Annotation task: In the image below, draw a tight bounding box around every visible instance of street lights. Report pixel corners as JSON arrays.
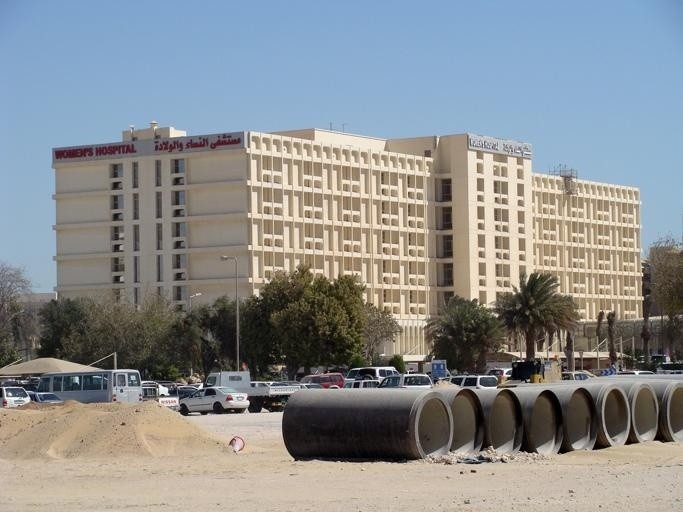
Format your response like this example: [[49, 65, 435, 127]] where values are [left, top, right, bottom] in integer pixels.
[[189, 291, 202, 311], [219, 254, 241, 372], [578, 350, 583, 371]]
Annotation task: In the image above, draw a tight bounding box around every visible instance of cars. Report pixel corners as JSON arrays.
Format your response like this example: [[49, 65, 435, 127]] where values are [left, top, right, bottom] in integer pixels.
[[178, 385, 251, 416]]
